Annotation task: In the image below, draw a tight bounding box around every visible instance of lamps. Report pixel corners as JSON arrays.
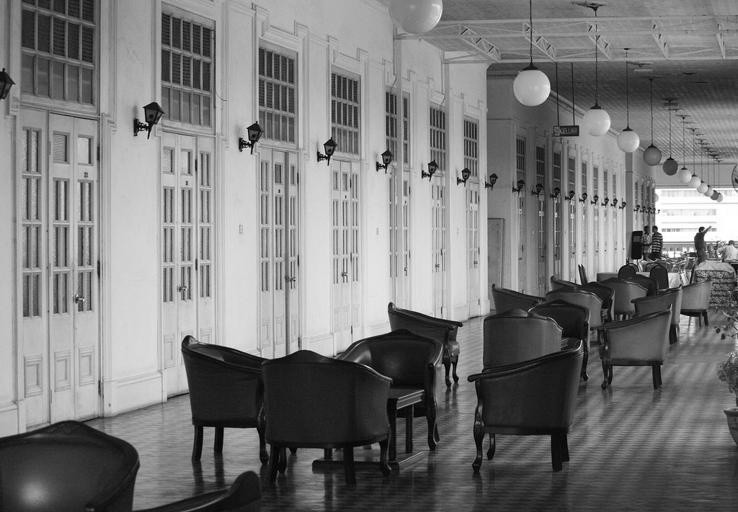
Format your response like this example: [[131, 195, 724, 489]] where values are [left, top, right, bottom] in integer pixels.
[[237, 121, 265, 157], [316, 137, 338, 167], [616, 51, 724, 203], [387, 1, 445, 35], [375, 150, 394, 175], [420, 159, 439, 183], [0, 68, 16, 101], [512, 0, 552, 109], [132, 101, 165, 141], [583, 9, 612, 139], [456, 167, 656, 216]]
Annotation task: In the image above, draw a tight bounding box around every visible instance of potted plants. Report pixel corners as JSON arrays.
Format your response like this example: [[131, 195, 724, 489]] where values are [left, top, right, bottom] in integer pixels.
[[713, 285, 737, 448]]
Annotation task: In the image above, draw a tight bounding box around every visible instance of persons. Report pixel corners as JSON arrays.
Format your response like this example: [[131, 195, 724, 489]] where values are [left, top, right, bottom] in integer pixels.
[[722, 240, 738, 262], [651, 226, 663, 260], [694, 226, 712, 263], [639, 226, 653, 260]]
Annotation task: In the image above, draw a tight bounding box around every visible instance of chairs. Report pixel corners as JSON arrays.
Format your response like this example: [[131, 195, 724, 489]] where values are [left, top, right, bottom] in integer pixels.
[[141, 469, 264, 512], [0, 416, 140, 512]]
